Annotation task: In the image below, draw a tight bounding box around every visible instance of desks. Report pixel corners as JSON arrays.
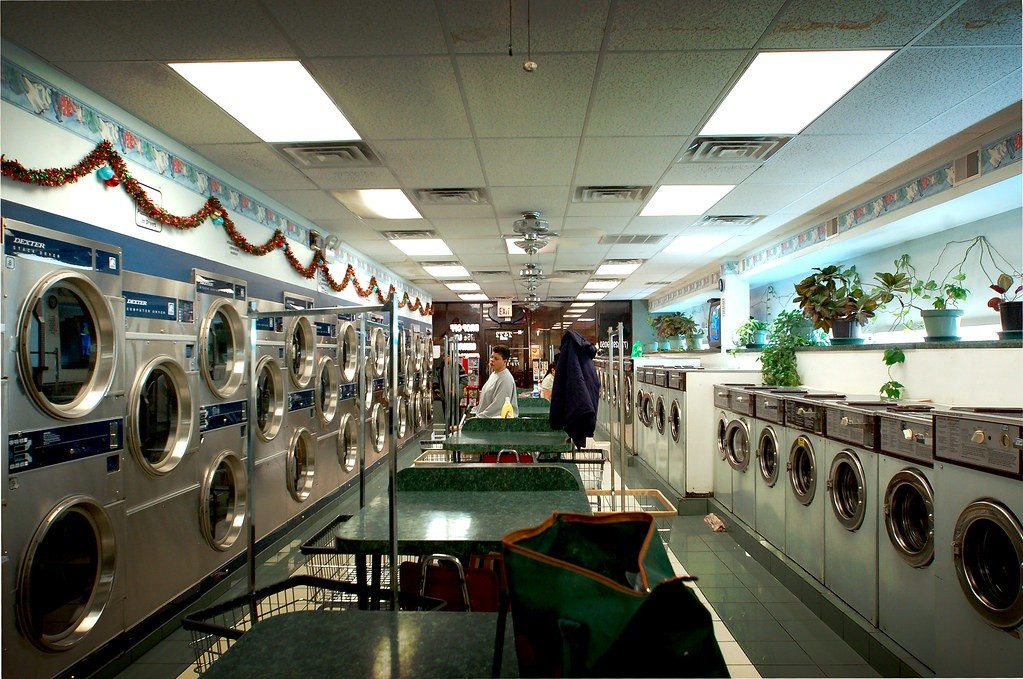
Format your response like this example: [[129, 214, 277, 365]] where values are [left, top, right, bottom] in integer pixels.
[[197, 610, 521, 679], [517, 398, 551, 419], [443, 418, 572, 463], [335, 463, 593, 611]]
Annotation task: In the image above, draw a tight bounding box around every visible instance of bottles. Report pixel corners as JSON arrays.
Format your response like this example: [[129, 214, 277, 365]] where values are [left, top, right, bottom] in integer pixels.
[[631, 341, 642, 357], [532, 387, 539, 398], [501, 397, 515, 418]]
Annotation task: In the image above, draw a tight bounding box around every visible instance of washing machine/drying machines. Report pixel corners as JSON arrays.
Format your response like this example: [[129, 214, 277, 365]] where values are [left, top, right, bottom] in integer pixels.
[[283, 291, 322, 528], [669, 370, 687, 497], [1, 216, 123, 679], [122, 271, 201, 637], [322, 307, 340, 504], [933, 405, 1023, 678], [713, 384, 743, 514], [247, 296, 288, 545], [823, 403, 915, 629], [644, 367, 655, 472], [755, 393, 805, 556], [592, 358, 632, 455], [340, 320, 361, 487], [656, 368, 668, 483], [783, 398, 834, 586], [729, 389, 774, 532], [876, 411, 932, 672], [360, 321, 432, 475], [636, 367, 644, 462], [192, 267, 248, 577]]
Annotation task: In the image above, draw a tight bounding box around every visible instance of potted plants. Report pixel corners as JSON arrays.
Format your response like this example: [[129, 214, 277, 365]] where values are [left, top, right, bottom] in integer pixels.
[[646, 312, 705, 352], [755, 309, 829, 389], [793, 265, 912, 338], [920, 284, 967, 337], [987, 274, 1023, 330], [728, 318, 773, 358]]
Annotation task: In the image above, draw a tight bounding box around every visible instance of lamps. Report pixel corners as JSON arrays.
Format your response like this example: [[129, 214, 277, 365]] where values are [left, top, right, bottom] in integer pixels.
[[523, 293, 540, 312], [518, 263, 543, 292], [512, 211, 559, 256], [321, 235, 344, 264]]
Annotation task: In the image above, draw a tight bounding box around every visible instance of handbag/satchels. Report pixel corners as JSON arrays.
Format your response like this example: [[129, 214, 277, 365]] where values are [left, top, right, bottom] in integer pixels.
[[501, 511, 729, 679]]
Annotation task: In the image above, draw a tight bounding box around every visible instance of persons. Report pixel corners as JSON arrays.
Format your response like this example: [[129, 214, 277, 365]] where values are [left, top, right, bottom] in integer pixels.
[[475, 345, 520, 419], [433, 350, 469, 435], [540, 361, 556, 403]]
